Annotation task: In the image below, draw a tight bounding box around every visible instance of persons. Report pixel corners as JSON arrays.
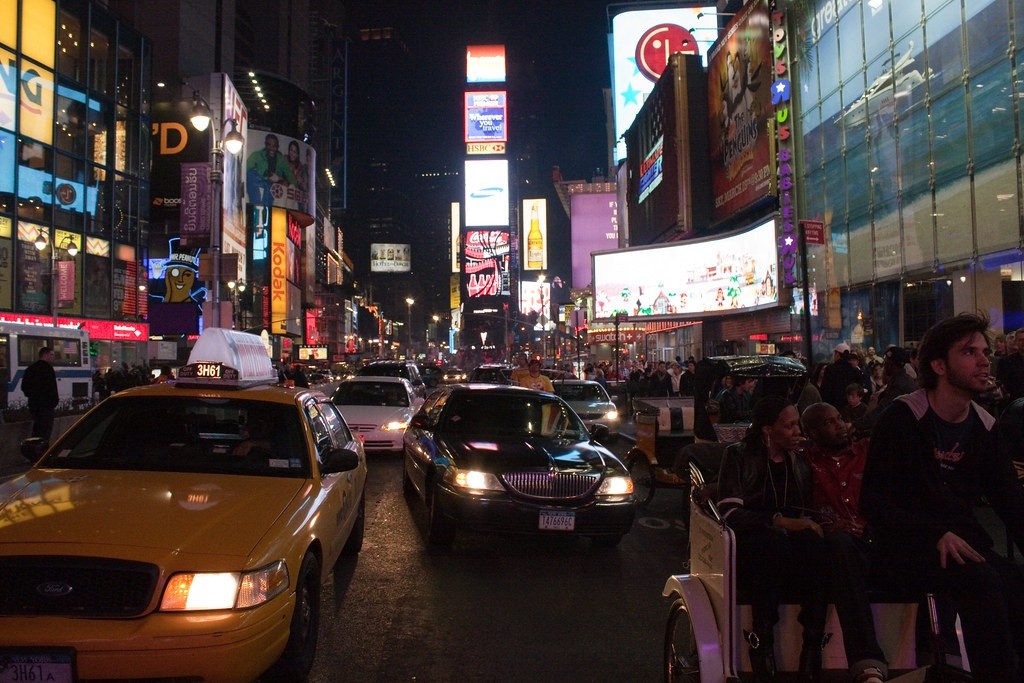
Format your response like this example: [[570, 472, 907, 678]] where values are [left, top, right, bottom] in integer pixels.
[[21, 347, 59, 441], [858, 313, 1024, 683], [512, 353, 555, 394], [716, 398, 890, 683], [585, 356, 705, 400], [716, 324, 1024, 427], [231, 405, 299, 457], [92, 362, 174, 392], [247, 134, 311, 192], [293, 365, 309, 388]]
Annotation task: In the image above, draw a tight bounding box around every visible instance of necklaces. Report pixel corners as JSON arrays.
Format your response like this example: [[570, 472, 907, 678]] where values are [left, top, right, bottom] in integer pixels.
[[768, 455, 788, 506], [530, 373, 540, 378]]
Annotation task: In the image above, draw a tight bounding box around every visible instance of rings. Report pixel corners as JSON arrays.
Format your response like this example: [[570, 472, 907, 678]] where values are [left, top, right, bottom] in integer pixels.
[[851, 528, 855, 532]]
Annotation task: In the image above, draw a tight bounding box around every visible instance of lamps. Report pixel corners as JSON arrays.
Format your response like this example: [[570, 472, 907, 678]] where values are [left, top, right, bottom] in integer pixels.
[[682, 39, 715, 46], [698, 12, 736, 20], [689, 28, 725, 34]]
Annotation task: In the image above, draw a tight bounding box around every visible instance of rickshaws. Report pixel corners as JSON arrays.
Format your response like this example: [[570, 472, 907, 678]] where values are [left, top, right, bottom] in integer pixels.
[[623, 394, 695, 506], [661, 443, 974, 683]]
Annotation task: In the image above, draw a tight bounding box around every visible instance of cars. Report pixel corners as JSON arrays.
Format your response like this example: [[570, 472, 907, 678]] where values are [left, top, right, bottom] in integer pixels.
[[328, 375, 426, 453], [284, 359, 370, 399], [548, 380, 621, 444], [415, 359, 470, 389], [402, 381, 636, 555], [0, 384, 368, 683]]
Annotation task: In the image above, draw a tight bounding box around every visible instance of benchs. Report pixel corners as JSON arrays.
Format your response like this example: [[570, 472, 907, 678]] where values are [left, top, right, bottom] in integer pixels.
[[631, 396, 695, 445], [681, 442, 881, 607]]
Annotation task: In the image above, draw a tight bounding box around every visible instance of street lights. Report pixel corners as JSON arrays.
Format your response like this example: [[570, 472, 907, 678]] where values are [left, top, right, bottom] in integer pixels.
[[35, 227, 78, 326], [188, 89, 246, 328], [405, 298, 415, 359]]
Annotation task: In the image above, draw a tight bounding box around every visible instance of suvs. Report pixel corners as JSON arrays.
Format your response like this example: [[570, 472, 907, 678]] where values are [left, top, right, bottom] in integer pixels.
[[470, 362, 515, 384], [356, 358, 426, 398]]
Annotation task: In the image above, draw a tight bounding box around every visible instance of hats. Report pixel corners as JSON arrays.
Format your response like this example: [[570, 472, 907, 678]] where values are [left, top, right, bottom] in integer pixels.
[[830, 343, 851, 354]]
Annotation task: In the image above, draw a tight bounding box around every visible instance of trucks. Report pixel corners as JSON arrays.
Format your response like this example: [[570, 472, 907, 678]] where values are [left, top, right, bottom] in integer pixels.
[[0, 322, 91, 415]]
